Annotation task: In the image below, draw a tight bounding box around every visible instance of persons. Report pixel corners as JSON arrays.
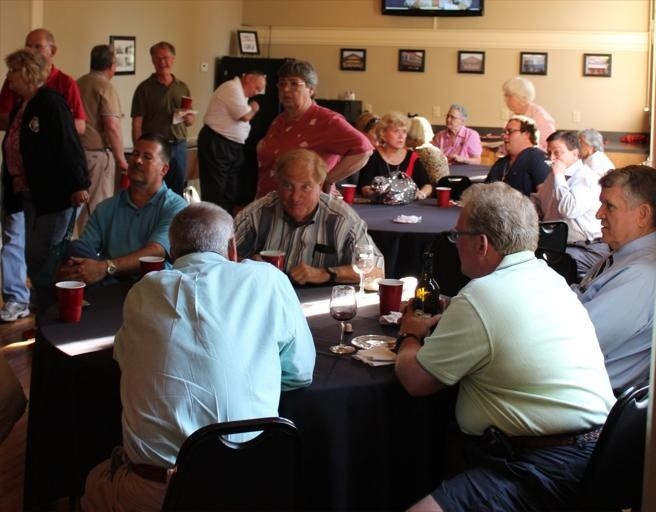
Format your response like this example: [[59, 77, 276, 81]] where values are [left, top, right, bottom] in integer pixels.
[[345, 76, 617, 282], [255, 56, 376, 204], [59, 131, 189, 319], [229, 150, 385, 285], [0, 26, 136, 323], [388, 181, 620, 509], [577, 166, 656, 398], [402, 1, 474, 13], [130, 42, 192, 198], [81, 202, 314, 511], [196, 71, 269, 213]]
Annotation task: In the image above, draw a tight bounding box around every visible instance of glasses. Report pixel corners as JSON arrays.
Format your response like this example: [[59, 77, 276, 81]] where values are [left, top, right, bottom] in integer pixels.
[[506, 128, 520, 135], [275, 80, 305, 89], [8, 67, 20, 73], [447, 229, 471, 243]]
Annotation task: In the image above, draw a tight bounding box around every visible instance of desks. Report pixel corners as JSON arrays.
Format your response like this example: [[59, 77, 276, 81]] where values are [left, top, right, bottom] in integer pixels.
[[31, 281, 133, 512], [340, 192, 466, 283], [448, 161, 493, 180], [280, 285, 453, 512]]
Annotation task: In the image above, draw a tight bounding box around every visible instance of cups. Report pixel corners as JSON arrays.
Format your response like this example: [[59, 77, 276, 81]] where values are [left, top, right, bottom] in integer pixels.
[[378, 277, 404, 319], [120, 171, 129, 189], [180, 96, 192, 111], [435, 186, 452, 207], [260, 249, 286, 273], [54, 280, 86, 323], [139, 256, 165, 277], [341, 183, 357, 206]]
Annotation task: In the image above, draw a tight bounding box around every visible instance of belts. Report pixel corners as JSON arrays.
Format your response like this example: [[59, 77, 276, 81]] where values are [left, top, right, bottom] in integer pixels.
[[512, 429, 599, 447], [130, 465, 166, 483]]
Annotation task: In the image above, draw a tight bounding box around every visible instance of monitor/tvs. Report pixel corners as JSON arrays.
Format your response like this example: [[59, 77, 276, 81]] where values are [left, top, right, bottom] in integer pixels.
[[380, 0, 485, 18]]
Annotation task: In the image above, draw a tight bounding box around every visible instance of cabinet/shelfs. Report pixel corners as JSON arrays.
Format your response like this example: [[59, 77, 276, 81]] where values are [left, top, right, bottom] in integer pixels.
[[214, 56, 365, 157]]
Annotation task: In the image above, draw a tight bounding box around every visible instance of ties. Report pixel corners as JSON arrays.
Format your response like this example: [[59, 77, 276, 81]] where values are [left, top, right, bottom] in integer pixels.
[[592, 256, 613, 278]]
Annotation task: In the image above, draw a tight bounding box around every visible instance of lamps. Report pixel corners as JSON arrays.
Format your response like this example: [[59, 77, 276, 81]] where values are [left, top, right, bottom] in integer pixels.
[[397, 48, 426, 72]]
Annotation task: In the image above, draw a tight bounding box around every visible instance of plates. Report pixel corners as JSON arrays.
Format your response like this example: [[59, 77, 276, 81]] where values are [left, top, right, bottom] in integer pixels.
[[352, 333, 398, 350]]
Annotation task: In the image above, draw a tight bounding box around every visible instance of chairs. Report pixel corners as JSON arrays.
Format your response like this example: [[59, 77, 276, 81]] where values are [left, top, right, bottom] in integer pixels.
[[433, 173, 474, 202], [535, 219, 581, 284], [566, 374, 650, 510], [161, 416, 316, 512]]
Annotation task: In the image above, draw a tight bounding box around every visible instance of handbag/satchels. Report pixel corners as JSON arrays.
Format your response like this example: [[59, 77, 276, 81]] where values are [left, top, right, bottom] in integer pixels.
[[46, 202, 90, 289], [369, 171, 418, 206]]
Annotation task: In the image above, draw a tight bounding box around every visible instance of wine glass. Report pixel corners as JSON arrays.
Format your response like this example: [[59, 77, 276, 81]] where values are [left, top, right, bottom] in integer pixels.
[[328, 284, 359, 356], [353, 242, 377, 301]]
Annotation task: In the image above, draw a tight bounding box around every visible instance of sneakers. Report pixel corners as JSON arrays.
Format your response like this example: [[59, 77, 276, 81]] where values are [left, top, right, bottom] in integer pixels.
[[0, 299, 30, 322]]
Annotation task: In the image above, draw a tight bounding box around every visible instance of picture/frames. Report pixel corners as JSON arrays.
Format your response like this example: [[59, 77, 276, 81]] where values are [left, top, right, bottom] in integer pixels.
[[109, 34, 137, 76], [457, 50, 486, 74], [582, 52, 612, 78], [235, 29, 259, 56], [519, 50, 548, 76], [339, 47, 367, 71]]
